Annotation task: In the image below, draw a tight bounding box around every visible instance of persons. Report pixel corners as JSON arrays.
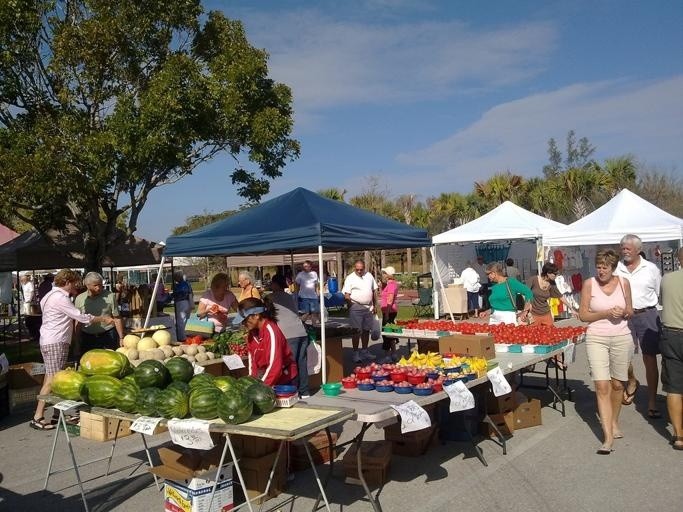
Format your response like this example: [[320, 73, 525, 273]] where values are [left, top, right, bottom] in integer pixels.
[[342, 260, 379, 363], [660, 247, 683, 450], [378, 266, 399, 364], [612, 234, 662, 418], [473, 256, 489, 311], [459, 261, 482, 318], [479, 261, 534, 325], [502, 258, 522, 281], [526, 262, 579, 360], [579, 249, 635, 454]]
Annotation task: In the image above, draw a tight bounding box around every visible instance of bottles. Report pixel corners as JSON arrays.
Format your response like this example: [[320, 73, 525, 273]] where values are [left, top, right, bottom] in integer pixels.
[[328, 277, 338, 294]]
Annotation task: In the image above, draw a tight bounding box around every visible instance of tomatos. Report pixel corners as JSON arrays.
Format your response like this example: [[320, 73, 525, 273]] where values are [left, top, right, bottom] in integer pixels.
[[397, 318, 586, 346]]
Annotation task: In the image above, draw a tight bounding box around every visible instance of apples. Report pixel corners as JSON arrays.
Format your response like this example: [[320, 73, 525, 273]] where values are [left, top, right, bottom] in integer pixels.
[[342, 362, 448, 390]]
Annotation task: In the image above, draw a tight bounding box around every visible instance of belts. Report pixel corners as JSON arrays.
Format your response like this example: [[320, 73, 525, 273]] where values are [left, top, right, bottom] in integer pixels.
[[633, 306, 656, 314]]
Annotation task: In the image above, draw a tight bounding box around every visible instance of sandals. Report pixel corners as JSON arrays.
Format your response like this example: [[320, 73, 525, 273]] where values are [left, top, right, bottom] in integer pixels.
[[669, 435, 683, 450], [29, 415, 60, 431]]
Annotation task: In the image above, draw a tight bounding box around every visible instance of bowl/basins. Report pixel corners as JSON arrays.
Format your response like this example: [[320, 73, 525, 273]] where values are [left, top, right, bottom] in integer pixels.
[[272, 385, 299, 393], [380, 318, 419, 334], [341, 352, 478, 396], [319, 383, 343, 396]]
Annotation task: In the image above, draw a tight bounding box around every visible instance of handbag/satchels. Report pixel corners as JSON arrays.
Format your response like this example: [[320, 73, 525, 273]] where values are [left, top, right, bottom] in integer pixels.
[[184, 312, 215, 338], [515, 309, 535, 326]]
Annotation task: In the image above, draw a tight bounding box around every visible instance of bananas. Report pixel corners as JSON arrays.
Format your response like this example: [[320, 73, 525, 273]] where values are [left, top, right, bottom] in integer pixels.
[[399, 351, 487, 372]]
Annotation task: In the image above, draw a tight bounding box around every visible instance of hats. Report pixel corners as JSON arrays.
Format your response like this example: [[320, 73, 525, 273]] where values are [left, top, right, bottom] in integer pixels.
[[232, 306, 265, 325], [381, 266, 396, 275]]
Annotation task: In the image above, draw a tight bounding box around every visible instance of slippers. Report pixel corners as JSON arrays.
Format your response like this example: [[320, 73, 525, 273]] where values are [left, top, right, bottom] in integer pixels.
[[613, 431, 625, 439], [648, 408, 663, 418], [622, 378, 640, 405], [596, 444, 612, 455]]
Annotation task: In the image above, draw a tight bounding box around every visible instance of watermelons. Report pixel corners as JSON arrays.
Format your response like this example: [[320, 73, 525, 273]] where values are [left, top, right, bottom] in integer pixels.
[[50, 349, 276, 425]]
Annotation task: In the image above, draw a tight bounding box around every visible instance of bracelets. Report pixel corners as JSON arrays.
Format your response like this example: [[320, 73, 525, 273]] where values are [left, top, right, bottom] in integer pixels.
[[570, 306, 574, 311], [523, 309, 529, 313]]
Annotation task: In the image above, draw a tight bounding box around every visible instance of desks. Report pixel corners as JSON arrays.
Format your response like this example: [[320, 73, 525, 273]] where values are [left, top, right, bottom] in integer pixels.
[[31, 304, 589, 511], [323, 291, 346, 312], [440, 287, 469, 321]]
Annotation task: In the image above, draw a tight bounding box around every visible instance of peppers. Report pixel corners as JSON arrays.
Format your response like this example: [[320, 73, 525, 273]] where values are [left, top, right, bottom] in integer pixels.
[[202, 331, 232, 359]]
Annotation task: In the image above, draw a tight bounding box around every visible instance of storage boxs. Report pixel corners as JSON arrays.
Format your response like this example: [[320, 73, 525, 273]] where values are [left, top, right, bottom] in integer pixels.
[[6, 361, 45, 407]]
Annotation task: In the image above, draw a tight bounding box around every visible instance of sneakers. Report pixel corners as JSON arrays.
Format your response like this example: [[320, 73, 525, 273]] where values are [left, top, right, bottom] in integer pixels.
[[352, 351, 362, 363], [359, 348, 377, 360], [377, 354, 400, 364]]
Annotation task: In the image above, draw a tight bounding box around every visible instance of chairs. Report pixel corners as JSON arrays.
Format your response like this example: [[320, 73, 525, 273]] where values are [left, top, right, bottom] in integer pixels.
[[412, 288, 433, 317]]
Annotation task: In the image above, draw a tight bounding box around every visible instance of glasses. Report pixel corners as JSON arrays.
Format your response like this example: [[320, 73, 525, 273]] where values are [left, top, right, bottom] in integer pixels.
[[355, 268, 364, 272]]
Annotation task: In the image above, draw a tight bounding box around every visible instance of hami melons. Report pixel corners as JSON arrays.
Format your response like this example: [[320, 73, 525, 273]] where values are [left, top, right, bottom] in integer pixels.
[[115, 330, 213, 367]]
